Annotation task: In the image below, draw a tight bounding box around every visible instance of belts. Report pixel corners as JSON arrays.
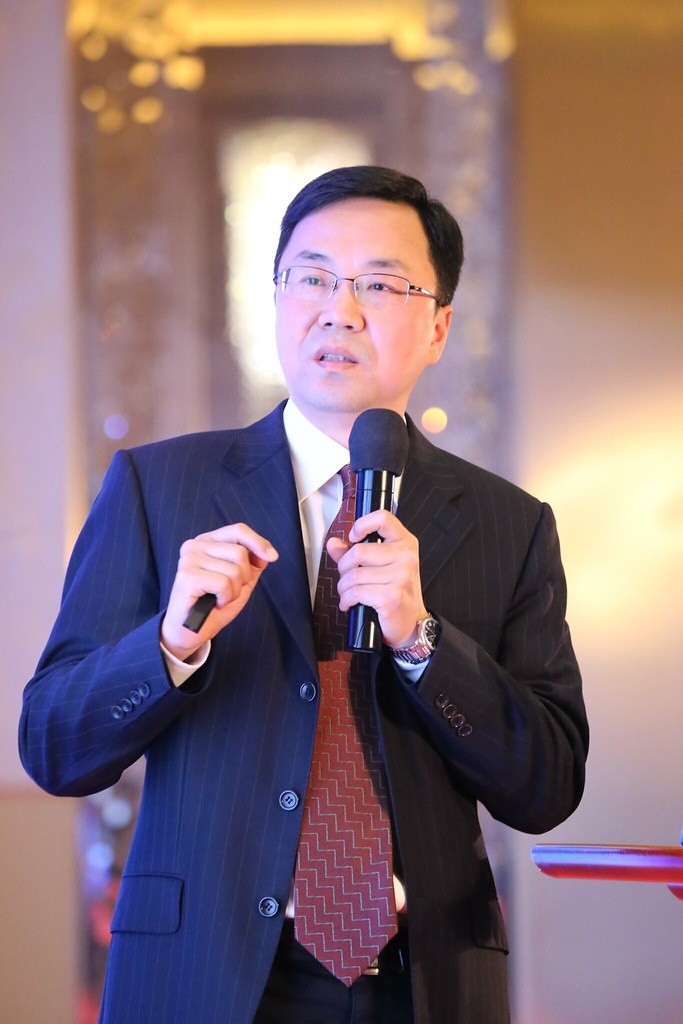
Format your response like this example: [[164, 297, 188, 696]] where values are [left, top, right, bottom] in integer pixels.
[[273, 934, 406, 977]]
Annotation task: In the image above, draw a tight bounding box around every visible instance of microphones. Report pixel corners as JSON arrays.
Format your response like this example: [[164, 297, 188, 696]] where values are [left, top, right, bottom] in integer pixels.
[[342, 408, 410, 652]]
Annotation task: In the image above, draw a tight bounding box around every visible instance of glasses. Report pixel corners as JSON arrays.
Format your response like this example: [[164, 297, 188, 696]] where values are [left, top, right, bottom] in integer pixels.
[[273, 265, 441, 310]]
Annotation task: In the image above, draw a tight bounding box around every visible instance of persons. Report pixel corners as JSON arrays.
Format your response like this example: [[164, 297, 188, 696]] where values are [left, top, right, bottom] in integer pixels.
[[17, 164, 590, 1024]]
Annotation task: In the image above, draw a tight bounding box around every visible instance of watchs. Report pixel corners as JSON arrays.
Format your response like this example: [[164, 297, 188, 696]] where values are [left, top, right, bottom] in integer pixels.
[[388, 613, 441, 665]]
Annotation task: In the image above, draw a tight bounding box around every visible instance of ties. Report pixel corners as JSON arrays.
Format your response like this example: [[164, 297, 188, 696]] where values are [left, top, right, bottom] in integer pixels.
[[293, 464, 398, 987]]
